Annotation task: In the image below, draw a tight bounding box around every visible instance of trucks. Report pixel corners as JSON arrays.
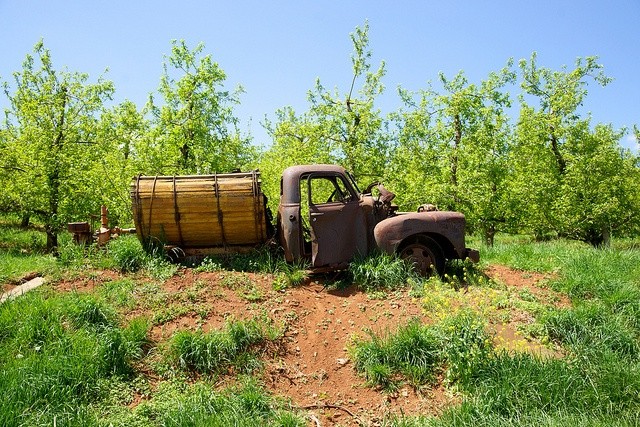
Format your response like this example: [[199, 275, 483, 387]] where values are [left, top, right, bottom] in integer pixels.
[[131, 163, 479, 282]]
